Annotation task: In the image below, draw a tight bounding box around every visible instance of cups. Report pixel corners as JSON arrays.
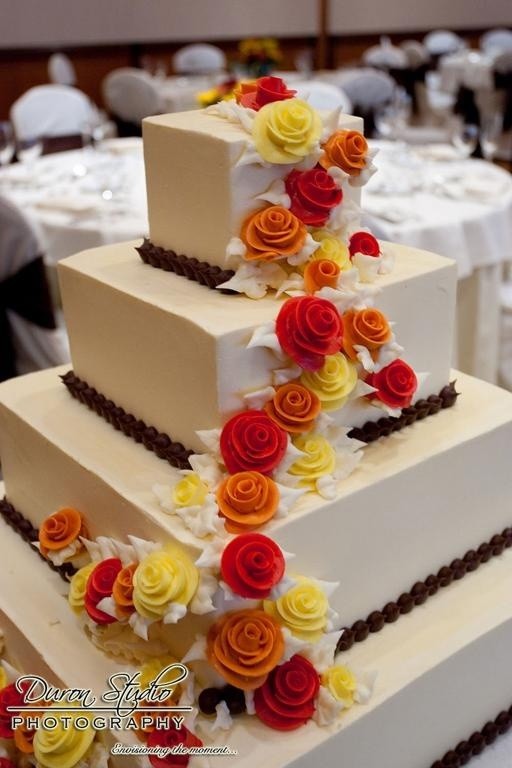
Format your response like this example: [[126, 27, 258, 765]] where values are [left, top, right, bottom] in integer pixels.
[[449, 124, 479, 160]]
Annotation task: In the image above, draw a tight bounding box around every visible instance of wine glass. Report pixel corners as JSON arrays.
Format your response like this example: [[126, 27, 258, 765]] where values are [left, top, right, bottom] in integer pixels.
[[0, 121, 46, 188]]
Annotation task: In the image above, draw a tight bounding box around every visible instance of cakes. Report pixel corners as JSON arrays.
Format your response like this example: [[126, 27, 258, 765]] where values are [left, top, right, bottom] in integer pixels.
[[0, 74, 512, 767]]
